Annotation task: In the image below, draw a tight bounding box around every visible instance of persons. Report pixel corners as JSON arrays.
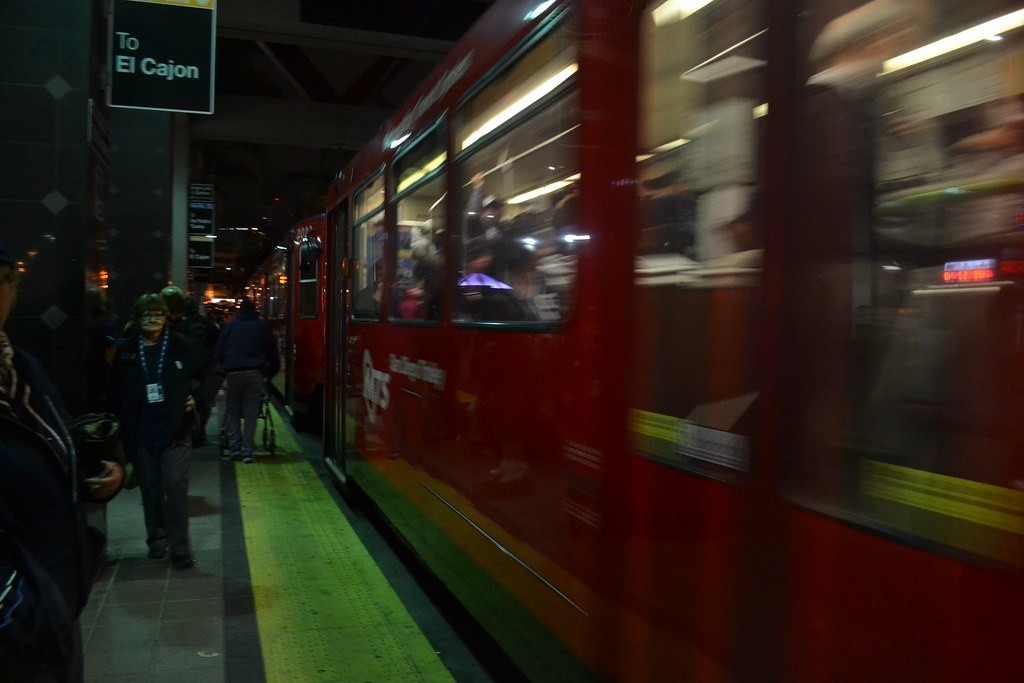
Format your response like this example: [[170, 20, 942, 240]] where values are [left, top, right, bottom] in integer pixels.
[[355, 170, 581, 319], [0, 246, 126, 683], [111, 285, 280, 567]]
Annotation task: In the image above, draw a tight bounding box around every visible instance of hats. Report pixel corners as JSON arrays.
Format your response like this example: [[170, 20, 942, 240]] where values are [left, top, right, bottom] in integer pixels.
[[240, 301, 255, 311], [0, 243, 14, 270]]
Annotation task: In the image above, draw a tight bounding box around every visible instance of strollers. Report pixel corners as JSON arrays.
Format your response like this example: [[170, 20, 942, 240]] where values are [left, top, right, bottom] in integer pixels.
[[220, 377, 276, 457]]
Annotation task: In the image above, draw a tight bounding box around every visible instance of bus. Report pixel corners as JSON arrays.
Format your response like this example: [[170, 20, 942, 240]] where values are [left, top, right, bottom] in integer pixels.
[[245, 1, 1024, 683]]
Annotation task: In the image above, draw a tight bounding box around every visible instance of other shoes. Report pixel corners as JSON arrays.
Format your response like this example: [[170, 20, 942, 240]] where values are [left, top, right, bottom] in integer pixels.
[[231, 446, 241, 458], [242, 451, 253, 462], [145, 542, 170, 563], [169, 549, 196, 566]]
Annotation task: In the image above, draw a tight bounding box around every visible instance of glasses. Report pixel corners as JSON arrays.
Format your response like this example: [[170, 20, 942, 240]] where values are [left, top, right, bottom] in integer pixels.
[[140, 313, 166, 320], [0, 270, 16, 284]]
